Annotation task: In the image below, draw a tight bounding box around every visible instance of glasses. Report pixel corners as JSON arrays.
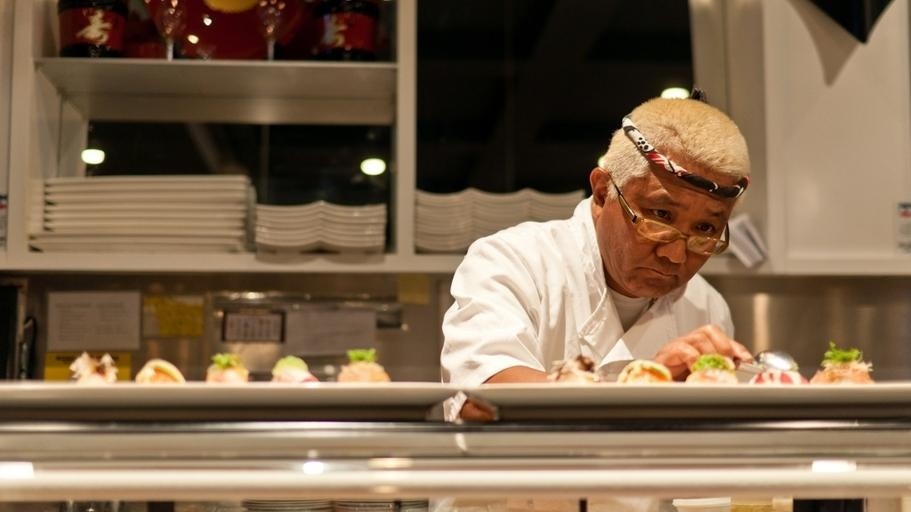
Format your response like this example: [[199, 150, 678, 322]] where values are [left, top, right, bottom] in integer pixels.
[[606, 172, 730, 256]]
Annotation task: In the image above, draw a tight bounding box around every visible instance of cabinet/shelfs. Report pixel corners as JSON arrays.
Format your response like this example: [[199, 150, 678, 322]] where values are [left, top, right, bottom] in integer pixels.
[[0, 1, 911, 278]]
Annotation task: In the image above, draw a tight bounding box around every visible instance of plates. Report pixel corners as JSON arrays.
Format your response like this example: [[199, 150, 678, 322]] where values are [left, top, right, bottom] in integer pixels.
[[454, 382, 911, 425], [413, 185, 585, 255], [26, 174, 254, 254], [0, 382, 461, 423], [251, 200, 388, 257]]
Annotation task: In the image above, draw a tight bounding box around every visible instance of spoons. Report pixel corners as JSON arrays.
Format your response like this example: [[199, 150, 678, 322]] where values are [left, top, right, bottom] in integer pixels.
[[735, 349, 794, 371]]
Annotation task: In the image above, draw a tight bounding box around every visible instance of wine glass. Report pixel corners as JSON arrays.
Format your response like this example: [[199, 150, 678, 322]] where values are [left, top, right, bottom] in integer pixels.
[[257, 0, 287, 61], [153, 0, 188, 60]]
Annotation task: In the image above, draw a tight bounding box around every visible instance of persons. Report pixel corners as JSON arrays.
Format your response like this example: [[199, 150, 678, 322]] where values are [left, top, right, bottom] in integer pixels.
[[438, 96, 753, 420]]
